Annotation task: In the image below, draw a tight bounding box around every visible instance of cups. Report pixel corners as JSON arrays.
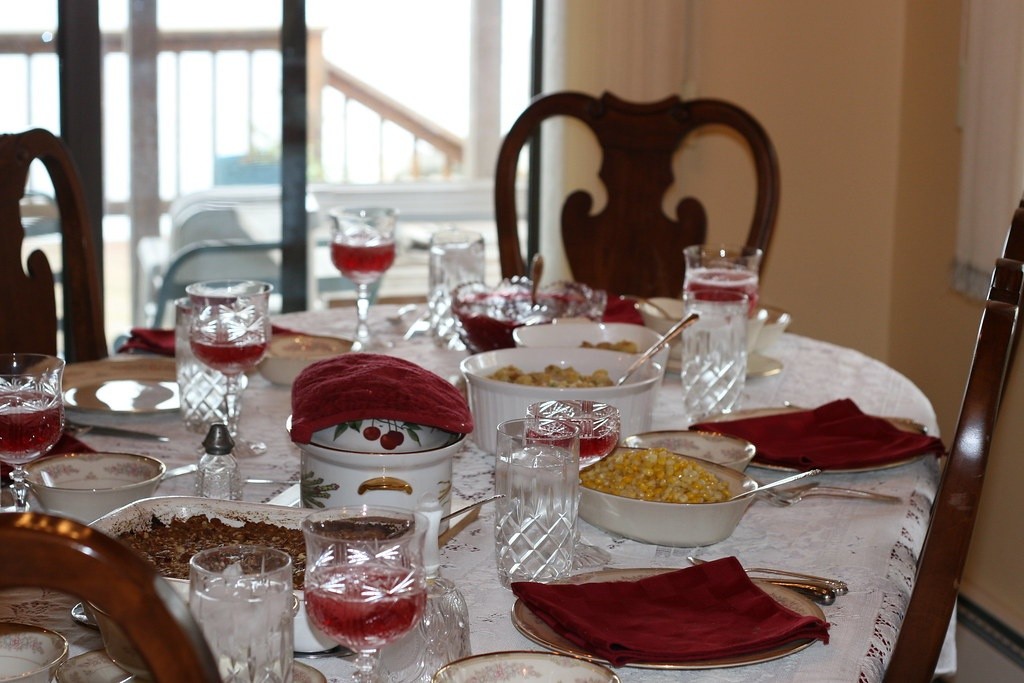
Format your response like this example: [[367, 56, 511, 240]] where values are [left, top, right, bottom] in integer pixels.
[[494, 415, 580, 590], [682, 244, 763, 325], [175, 301, 246, 437], [187, 544, 296, 683], [680, 287, 749, 426], [426, 230, 485, 355]]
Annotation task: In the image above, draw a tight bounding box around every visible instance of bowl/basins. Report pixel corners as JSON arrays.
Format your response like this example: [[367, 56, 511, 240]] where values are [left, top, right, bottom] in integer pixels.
[[84, 577, 190, 683], [619, 429, 757, 474], [0, 621, 70, 683], [512, 320, 670, 372], [449, 280, 608, 354], [284, 412, 470, 537], [578, 445, 758, 548], [21, 452, 167, 523], [640, 298, 791, 379], [459, 346, 664, 456], [257, 333, 354, 386]]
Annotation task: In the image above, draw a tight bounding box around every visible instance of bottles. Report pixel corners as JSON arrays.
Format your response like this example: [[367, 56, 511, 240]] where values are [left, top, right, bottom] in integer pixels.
[[193, 422, 244, 502], [367, 489, 472, 683]]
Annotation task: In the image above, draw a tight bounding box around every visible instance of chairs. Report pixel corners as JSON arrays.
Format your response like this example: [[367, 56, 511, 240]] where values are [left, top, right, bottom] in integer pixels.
[[493, 87, 789, 307], [0, 127, 109, 363], [131, 184, 283, 329], [880, 195, 1024, 683], [0, 513, 212, 683]]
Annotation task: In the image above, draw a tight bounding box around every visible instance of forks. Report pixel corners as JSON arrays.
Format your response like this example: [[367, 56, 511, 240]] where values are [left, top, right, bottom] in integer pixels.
[[748, 475, 902, 505]]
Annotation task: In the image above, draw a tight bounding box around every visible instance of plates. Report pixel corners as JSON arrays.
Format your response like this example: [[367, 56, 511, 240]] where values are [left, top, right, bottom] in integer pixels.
[[699, 406, 929, 475], [63, 379, 186, 438], [85, 494, 313, 592], [54, 648, 150, 683], [511, 566, 827, 670], [432, 649, 620, 683]]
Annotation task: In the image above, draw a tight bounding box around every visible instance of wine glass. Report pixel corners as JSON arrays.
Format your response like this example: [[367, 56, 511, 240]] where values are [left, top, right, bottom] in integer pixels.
[[185, 280, 275, 456], [331, 221, 398, 355], [522, 400, 621, 568], [0, 349, 66, 513], [301, 505, 428, 683]]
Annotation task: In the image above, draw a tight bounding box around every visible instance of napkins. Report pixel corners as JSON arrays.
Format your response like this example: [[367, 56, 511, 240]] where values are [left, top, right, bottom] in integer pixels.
[[512, 554, 835, 668], [293, 350, 474, 441], [117, 326, 283, 357], [692, 396, 945, 469]]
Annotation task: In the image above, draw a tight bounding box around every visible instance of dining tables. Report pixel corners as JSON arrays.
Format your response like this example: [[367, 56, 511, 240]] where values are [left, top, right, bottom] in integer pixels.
[[0, 303, 960, 683]]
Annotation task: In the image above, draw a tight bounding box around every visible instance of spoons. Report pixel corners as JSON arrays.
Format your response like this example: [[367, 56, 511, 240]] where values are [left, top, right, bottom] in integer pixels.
[[687, 555, 849, 597]]
